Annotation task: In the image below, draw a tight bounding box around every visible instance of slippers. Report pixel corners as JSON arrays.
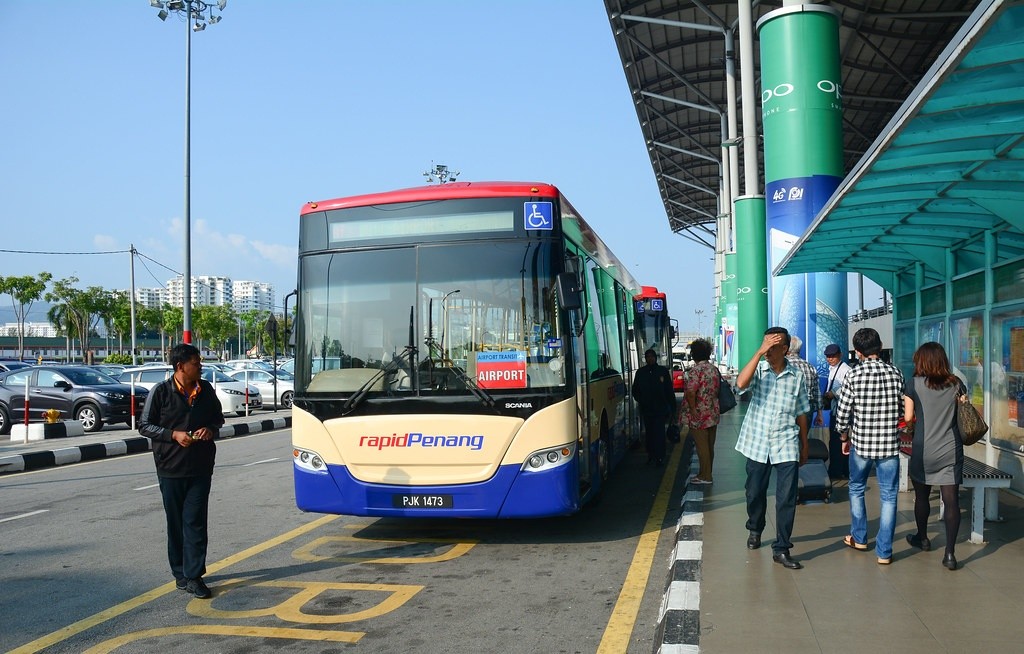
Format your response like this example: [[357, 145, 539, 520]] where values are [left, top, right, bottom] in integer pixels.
[[691, 477, 713, 484]]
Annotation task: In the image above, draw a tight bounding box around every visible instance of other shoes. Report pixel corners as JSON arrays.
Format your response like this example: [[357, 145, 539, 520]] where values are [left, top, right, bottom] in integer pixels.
[[878, 556, 892, 564], [844, 535, 867, 550], [657, 456, 664, 465]]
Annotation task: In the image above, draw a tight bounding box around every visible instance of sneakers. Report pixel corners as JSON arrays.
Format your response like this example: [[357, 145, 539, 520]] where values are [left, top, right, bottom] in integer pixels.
[[176, 577, 188, 590], [187, 577, 211, 598]]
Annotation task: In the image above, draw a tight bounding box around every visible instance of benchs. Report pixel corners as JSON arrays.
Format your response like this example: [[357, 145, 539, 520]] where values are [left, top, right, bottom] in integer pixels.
[[898, 447, 1013, 545]]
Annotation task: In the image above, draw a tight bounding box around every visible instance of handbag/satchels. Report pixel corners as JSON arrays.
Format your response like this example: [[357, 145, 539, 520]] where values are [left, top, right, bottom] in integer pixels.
[[955, 380, 988, 446], [822, 395, 832, 410], [716, 367, 737, 414], [667, 416, 681, 443]]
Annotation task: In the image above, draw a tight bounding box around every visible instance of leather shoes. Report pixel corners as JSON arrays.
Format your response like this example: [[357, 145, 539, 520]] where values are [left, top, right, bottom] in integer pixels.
[[773, 552, 801, 569], [906, 533, 931, 550], [747, 531, 761, 549], [943, 553, 957, 570]]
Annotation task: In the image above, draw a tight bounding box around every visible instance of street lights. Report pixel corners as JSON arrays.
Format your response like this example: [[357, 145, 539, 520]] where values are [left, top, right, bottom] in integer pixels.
[[147, 0, 226, 349]]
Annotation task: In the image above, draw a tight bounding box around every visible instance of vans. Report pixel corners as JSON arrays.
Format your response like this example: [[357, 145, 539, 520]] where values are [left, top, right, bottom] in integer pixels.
[[275, 356, 342, 386]]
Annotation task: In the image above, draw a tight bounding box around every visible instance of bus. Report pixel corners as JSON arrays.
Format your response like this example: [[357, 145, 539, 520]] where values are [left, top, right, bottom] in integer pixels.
[[263, 179, 646, 522], [633, 286, 680, 424]]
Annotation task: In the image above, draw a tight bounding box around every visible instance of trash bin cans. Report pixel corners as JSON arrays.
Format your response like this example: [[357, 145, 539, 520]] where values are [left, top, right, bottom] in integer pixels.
[[87, 351, 95, 365], [1016, 393, 1024, 428], [34, 352, 40, 359], [730, 374, 747, 402]]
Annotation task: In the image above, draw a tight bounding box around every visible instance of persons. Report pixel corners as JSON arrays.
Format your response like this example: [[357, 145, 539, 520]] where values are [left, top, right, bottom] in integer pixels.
[[139, 344, 225, 597], [991, 352, 1006, 385], [734, 327, 810, 569], [824, 344, 853, 479], [972, 351, 983, 386], [786, 336, 823, 434], [904, 342, 968, 570], [632, 349, 677, 466], [835, 328, 906, 563], [678, 338, 720, 484]]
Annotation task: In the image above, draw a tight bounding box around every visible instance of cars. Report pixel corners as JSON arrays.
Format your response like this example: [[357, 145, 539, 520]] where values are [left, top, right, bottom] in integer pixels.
[[0, 364, 149, 436], [670, 339, 694, 392], [0, 356, 296, 425]]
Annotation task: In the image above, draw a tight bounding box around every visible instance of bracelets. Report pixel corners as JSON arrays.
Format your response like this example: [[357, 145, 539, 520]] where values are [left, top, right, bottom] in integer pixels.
[[841, 436, 849, 442]]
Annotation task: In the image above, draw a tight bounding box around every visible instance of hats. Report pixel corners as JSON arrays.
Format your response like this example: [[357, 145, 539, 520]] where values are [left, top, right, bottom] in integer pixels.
[[824, 344, 841, 356]]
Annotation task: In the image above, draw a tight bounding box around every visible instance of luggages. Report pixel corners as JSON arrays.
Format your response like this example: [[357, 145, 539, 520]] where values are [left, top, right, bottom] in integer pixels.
[[796, 458, 832, 503]]
